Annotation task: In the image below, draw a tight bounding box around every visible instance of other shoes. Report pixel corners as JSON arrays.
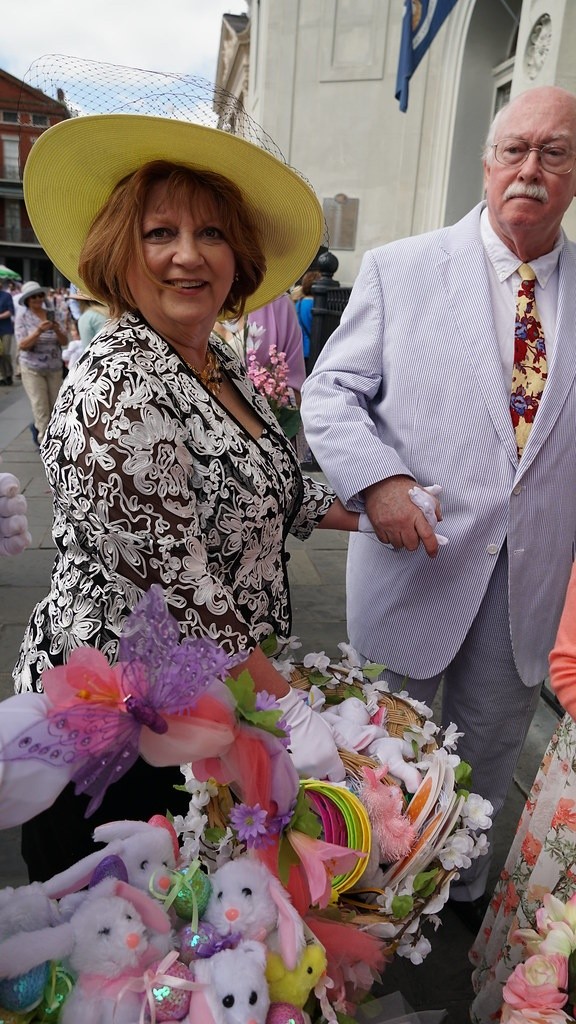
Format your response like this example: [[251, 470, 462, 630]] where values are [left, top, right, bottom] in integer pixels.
[[0, 380, 13, 386], [30, 423, 40, 446], [15, 373, 21, 377], [444, 890, 492, 939]]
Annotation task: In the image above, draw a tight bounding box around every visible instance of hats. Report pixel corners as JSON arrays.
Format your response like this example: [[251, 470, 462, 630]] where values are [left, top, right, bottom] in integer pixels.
[[65, 289, 96, 300], [18, 281, 48, 305], [23, 115, 323, 321]]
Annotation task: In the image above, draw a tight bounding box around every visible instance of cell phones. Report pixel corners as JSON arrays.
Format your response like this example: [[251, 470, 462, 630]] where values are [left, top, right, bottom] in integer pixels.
[[46, 310, 55, 323]]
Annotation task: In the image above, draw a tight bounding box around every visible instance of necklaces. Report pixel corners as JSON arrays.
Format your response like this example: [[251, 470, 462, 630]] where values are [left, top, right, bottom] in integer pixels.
[[182, 344, 224, 397]]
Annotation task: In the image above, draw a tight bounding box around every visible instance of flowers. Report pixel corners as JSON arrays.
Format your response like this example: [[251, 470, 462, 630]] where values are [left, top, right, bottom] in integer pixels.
[[214, 315, 300, 418], [173, 633, 494, 967]]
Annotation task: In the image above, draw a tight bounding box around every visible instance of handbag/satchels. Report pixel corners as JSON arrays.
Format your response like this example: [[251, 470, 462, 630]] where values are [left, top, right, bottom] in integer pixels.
[[63, 361, 69, 379]]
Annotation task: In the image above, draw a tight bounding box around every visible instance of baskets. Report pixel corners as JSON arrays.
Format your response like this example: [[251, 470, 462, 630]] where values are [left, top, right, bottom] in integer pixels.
[[202, 672, 468, 956]]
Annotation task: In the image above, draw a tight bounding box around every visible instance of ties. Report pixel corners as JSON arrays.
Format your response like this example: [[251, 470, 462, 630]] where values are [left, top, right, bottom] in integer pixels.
[[510, 263, 548, 462]]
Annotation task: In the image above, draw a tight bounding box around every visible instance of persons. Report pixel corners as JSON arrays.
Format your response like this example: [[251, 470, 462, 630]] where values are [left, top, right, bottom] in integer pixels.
[[0, 277, 110, 449], [230, 271, 320, 458], [298, 85, 576, 932], [24, 114, 450, 889]]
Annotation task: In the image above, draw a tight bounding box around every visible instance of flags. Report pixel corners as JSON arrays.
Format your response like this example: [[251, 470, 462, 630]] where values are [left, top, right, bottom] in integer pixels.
[[395, 0, 458, 113]]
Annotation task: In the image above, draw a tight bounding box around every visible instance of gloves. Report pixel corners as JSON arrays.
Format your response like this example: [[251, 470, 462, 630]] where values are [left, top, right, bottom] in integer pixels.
[[276, 684, 346, 782], [0, 473, 31, 557], [358, 484, 448, 545]]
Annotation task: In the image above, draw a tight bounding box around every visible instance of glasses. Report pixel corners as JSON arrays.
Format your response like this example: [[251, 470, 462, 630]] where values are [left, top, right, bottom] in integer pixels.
[[30, 293, 44, 299], [490, 138, 576, 175]]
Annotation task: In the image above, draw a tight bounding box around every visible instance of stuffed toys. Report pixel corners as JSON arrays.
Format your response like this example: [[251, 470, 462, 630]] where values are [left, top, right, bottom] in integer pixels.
[[0, 820, 327, 1024], [322, 698, 422, 794]]
